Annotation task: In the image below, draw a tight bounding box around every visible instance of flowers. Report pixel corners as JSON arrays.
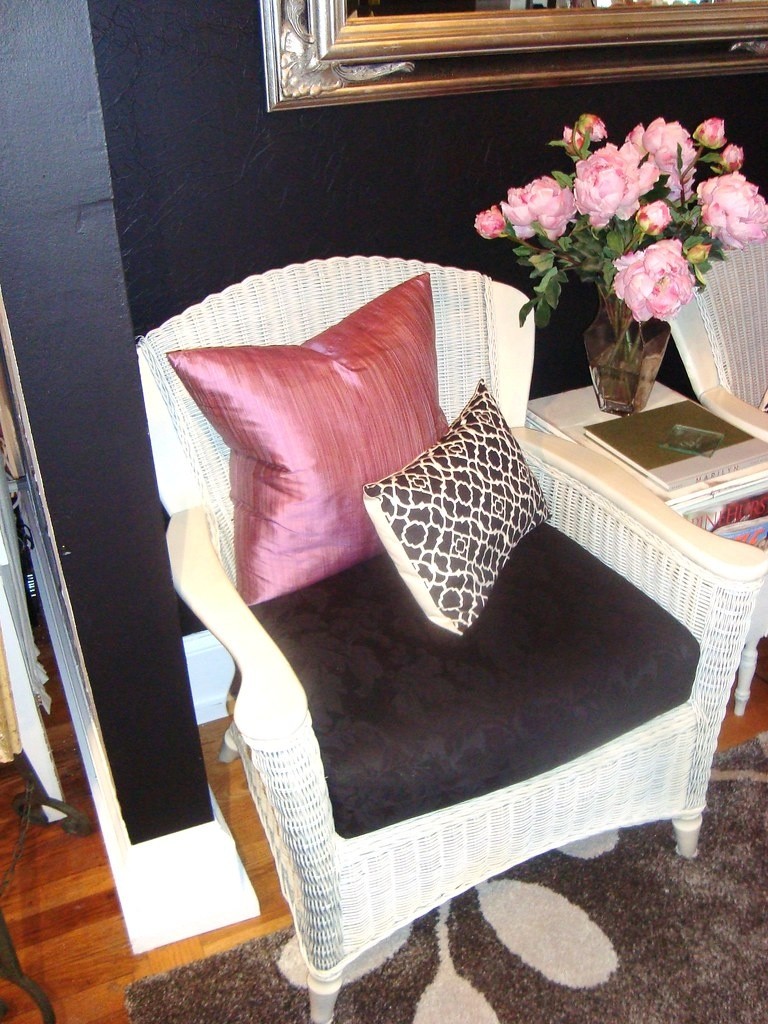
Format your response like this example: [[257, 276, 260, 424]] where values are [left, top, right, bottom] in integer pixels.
[[474, 114, 768, 405]]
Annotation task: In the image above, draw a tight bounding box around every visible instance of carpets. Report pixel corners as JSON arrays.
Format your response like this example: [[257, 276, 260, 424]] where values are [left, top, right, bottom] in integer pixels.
[[125, 730, 768, 1024]]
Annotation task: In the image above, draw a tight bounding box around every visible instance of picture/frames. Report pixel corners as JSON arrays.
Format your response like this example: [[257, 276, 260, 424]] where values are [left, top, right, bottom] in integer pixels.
[[260, 0, 768, 113]]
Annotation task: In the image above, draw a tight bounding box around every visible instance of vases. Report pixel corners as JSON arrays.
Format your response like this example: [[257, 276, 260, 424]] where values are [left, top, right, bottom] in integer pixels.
[[584, 289, 670, 417]]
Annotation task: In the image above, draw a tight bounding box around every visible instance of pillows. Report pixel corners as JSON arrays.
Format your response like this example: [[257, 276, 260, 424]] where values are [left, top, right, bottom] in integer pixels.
[[362, 378, 552, 637], [167, 273, 456, 604]]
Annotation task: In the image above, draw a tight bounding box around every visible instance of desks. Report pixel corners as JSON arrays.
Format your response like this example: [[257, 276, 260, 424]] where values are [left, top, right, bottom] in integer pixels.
[[527, 381, 768, 717]]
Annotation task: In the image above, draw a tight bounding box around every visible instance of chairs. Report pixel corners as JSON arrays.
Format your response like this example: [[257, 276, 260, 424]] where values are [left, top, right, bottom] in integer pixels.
[[137, 241, 768, 1024]]
